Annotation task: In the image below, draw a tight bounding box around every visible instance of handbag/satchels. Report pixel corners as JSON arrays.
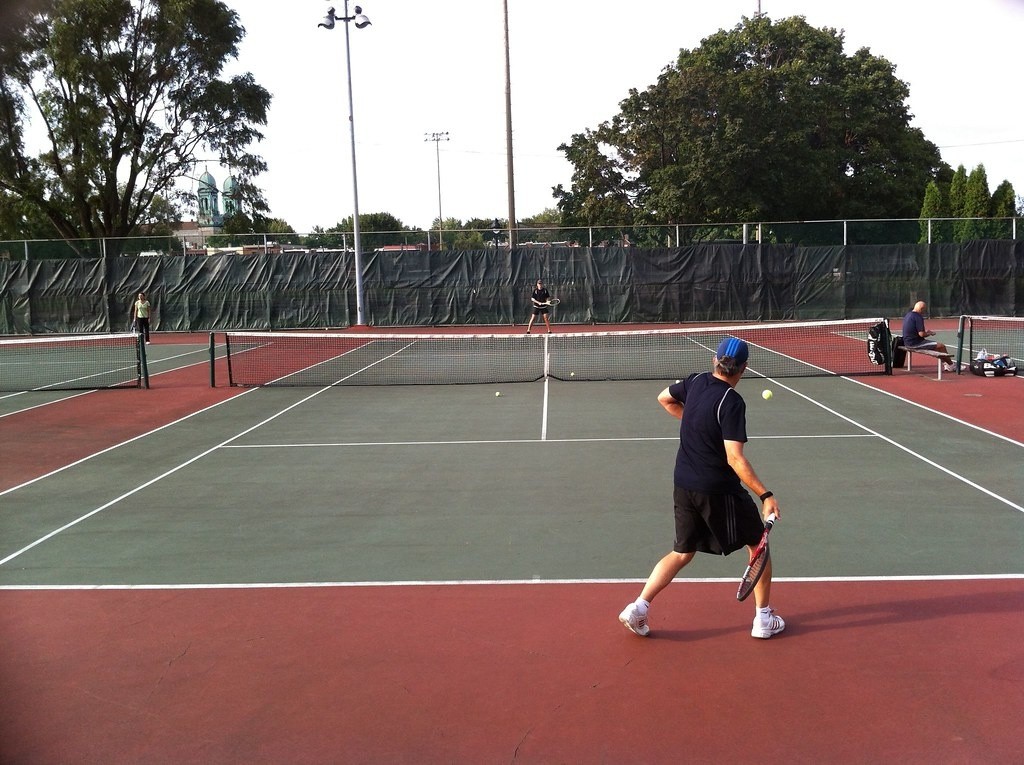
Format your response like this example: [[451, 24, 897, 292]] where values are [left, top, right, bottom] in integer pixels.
[[971, 349, 1018, 378]]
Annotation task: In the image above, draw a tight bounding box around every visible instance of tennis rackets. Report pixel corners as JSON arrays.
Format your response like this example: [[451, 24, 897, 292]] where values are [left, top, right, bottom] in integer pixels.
[[540, 299, 560, 306], [735, 509, 779, 601]]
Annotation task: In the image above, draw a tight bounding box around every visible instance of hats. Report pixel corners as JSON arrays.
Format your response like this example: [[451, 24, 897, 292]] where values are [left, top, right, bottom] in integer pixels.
[[716, 338, 749, 364], [537, 280, 543, 284]]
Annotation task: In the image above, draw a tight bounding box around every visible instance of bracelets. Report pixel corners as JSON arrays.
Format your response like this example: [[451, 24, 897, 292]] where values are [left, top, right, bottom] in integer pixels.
[[760, 492, 773, 502]]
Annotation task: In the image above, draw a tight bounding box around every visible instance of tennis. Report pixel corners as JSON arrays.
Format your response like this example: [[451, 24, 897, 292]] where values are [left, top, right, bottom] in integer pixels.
[[762, 389, 773, 400], [571, 372, 574, 376], [495, 391, 500, 396], [675, 380, 680, 384]]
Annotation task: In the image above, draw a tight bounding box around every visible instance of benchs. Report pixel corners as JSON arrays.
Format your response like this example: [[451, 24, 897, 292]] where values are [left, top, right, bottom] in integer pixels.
[[898, 346, 955, 380]]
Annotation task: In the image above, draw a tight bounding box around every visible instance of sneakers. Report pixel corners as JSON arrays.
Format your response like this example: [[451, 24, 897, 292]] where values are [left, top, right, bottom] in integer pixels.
[[944, 364, 948, 371], [618, 602, 650, 637], [948, 361, 967, 372], [751, 610, 786, 639]]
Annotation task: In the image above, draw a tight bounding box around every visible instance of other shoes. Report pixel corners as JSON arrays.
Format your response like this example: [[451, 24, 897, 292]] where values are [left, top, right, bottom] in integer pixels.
[[526, 330, 530, 334], [547, 330, 552, 333], [146, 342, 151, 345]]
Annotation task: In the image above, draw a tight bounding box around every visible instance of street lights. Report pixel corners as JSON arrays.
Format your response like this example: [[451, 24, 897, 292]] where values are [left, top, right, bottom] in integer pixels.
[[316, 1, 374, 326], [490, 218, 502, 249], [423, 130, 451, 250]]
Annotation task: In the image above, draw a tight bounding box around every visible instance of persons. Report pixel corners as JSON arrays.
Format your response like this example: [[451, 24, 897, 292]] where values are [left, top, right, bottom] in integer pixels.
[[133, 293, 151, 345], [619, 339, 787, 639], [903, 301, 967, 371], [527, 279, 552, 334]]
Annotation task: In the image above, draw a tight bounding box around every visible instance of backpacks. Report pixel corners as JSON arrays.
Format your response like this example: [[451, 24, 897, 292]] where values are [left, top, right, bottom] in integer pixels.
[[892, 336, 907, 368], [867, 316, 892, 365]]
